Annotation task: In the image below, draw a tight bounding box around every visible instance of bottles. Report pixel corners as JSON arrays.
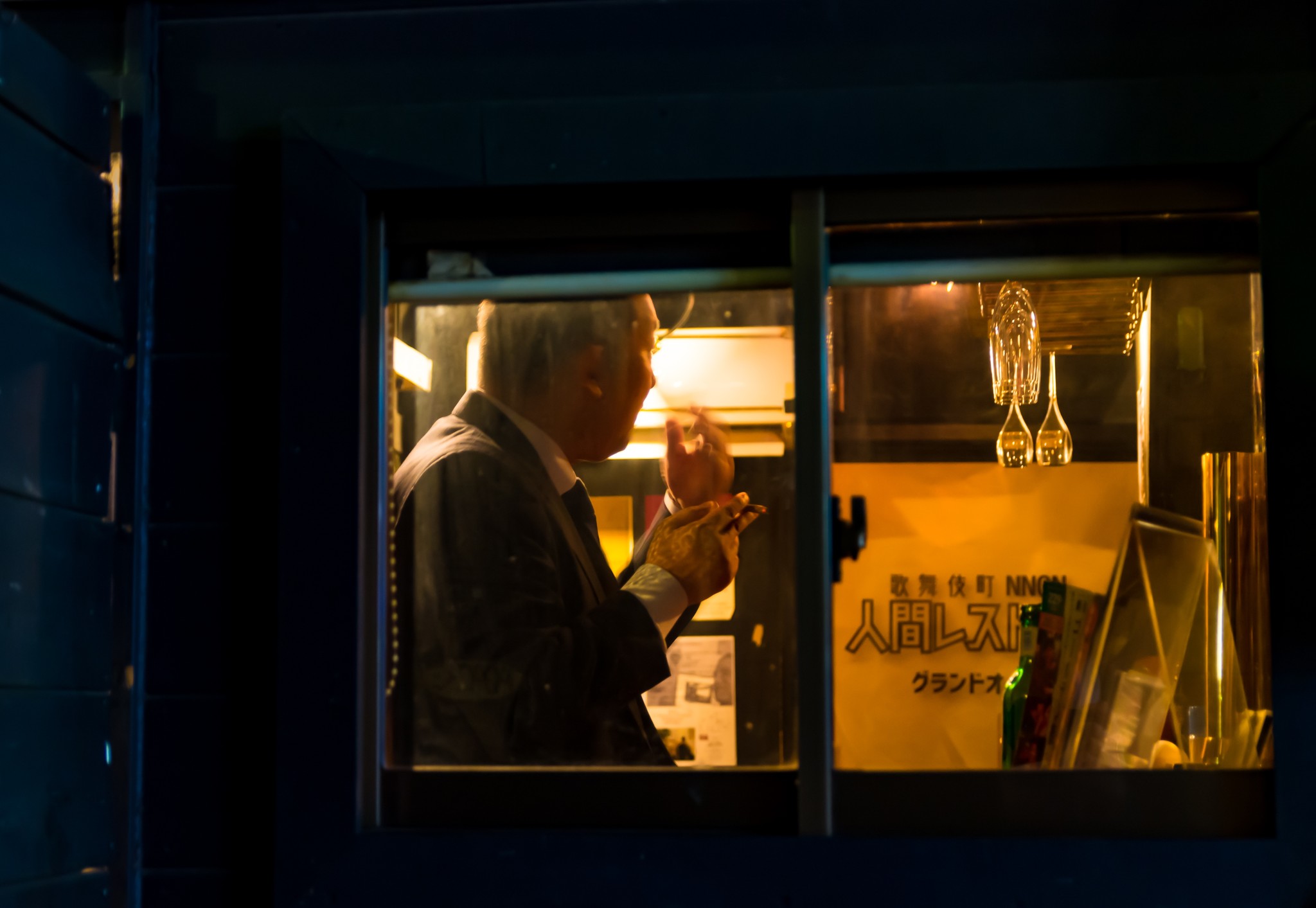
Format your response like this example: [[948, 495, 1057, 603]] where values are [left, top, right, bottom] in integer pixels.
[[1001, 604, 1040, 770]]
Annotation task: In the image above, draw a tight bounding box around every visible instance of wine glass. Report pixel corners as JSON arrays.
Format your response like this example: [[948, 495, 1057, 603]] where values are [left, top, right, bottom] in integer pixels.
[[1035, 344, 1073, 466]]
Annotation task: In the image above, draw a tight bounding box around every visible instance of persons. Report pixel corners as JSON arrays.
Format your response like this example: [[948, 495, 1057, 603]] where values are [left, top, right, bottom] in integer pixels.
[[389, 292, 766, 768]]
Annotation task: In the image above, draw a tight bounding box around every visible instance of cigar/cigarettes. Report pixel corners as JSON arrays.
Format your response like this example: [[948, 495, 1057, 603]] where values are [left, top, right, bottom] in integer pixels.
[[743, 505, 767, 513]]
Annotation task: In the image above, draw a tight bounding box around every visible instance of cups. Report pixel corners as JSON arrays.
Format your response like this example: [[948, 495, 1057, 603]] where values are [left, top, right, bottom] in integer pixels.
[[996, 405, 1034, 467], [988, 280, 1041, 405]]
